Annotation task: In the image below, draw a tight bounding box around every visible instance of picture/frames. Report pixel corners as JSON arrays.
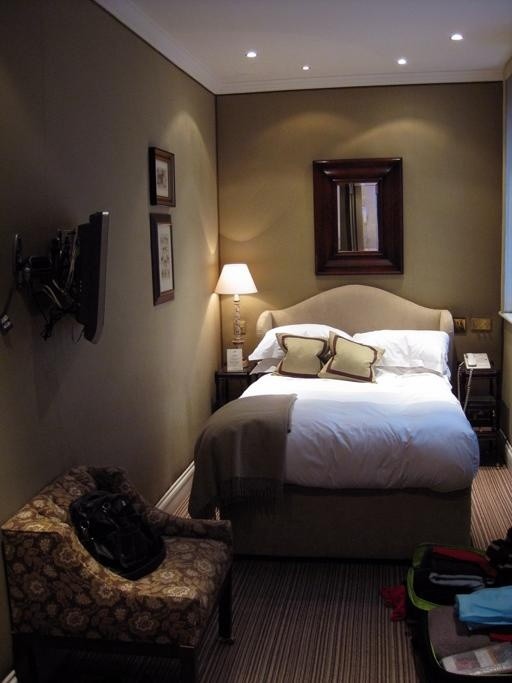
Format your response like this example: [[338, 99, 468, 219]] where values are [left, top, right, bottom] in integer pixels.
[[147, 144, 177, 207], [149, 212, 175, 306]]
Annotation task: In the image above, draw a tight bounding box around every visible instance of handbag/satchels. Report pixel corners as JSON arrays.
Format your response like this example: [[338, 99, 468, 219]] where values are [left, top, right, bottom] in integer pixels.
[[71, 490, 166, 581]]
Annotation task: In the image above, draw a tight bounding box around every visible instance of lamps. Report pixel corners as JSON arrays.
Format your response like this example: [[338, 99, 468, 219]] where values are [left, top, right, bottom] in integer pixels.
[[214, 263, 258, 368]]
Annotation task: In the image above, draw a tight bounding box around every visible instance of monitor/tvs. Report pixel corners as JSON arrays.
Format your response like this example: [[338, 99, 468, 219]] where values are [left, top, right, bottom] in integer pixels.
[[76, 211, 109, 344]]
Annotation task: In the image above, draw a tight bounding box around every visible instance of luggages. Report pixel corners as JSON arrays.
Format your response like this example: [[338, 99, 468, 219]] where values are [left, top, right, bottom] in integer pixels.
[[414, 605, 512, 683], [406, 542, 512, 624]]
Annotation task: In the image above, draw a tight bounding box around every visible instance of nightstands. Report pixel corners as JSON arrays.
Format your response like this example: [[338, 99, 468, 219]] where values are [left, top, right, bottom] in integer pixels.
[[214, 370, 257, 410], [454, 361, 502, 467]]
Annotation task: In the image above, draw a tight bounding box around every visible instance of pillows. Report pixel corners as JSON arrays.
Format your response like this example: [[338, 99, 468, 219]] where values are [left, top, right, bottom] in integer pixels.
[[349, 327, 450, 377], [318, 331, 385, 383], [242, 322, 347, 360], [276, 333, 328, 378], [245, 360, 308, 377]]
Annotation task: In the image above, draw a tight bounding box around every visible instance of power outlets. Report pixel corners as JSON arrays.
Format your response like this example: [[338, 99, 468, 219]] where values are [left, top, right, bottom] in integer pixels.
[[454, 317, 467, 336]]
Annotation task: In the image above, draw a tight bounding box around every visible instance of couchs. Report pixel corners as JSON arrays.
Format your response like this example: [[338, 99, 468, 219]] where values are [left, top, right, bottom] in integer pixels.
[[0, 462, 236, 683]]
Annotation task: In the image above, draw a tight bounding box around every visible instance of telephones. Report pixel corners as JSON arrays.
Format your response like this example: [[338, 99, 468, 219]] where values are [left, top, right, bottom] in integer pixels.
[[464, 352, 491, 371]]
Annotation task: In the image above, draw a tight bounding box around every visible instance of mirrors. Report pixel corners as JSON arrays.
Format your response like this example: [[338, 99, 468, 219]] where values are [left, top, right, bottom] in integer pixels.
[[314, 157, 405, 275]]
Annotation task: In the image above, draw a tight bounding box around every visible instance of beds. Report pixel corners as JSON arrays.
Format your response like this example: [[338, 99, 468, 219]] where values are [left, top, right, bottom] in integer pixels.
[[198, 284, 480, 559]]
